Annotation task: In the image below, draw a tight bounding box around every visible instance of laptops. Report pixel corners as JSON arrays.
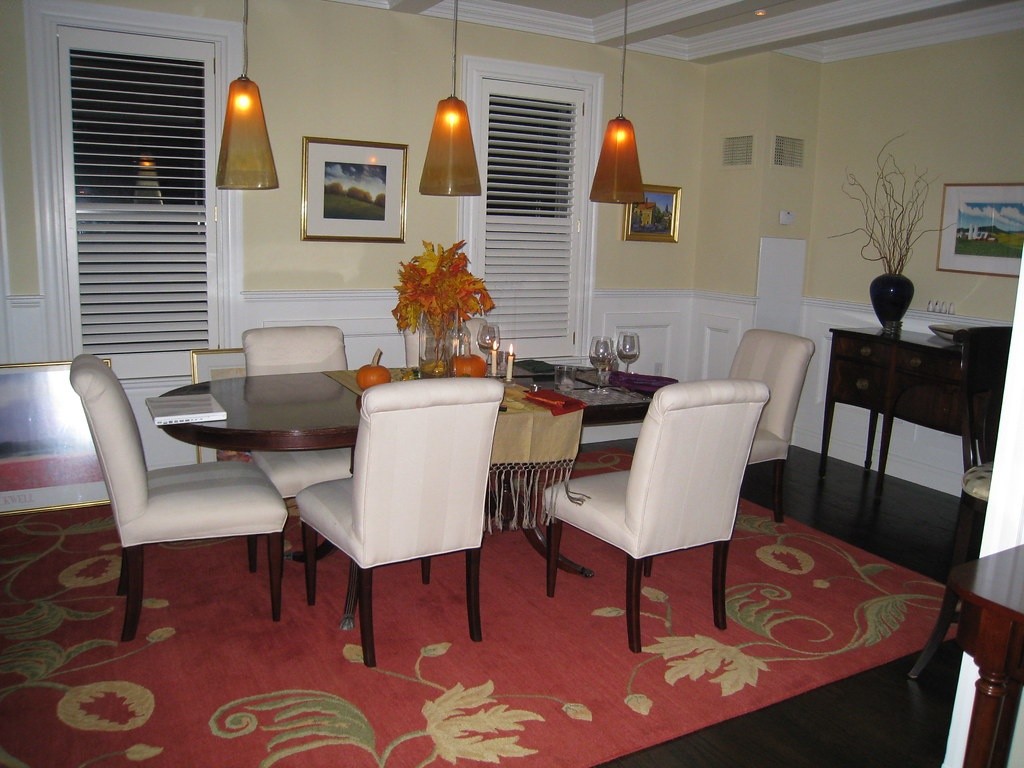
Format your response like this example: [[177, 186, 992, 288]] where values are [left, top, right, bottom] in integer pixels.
[[144, 393, 227, 425]]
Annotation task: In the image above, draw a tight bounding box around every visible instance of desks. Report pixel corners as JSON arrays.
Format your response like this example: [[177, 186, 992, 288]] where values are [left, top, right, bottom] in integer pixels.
[[950, 545, 1024, 768], [160, 365, 654, 630]]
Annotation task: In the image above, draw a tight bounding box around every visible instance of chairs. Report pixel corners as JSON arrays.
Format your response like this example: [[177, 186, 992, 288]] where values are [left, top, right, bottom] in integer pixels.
[[908, 326, 1013, 680], [296, 377, 504, 667], [70, 354, 288, 642], [727, 330, 815, 523], [403, 319, 489, 368], [242, 326, 352, 498], [546, 378, 770, 653]]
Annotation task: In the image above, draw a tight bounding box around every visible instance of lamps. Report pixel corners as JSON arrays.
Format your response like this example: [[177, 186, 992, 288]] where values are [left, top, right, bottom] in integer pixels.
[[589, 1, 646, 203], [418, 0, 482, 196], [216, 0, 280, 190]]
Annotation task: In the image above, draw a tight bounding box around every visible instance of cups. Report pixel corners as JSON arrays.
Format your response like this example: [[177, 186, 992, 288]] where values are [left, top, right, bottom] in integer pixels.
[[497, 351, 516, 375], [554, 365, 578, 392]]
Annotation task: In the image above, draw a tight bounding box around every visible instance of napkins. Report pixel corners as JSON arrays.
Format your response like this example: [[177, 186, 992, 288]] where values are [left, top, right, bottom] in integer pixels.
[[523, 389, 587, 416], [515, 360, 556, 373]]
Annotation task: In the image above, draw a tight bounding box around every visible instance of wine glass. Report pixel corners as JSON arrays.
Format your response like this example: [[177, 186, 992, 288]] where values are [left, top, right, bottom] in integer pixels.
[[476, 324, 500, 377], [617, 332, 640, 374], [589, 336, 618, 395]]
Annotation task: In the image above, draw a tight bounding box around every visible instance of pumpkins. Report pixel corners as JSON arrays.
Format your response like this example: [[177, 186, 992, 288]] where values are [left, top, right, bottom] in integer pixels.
[[356, 348, 390, 390], [448, 343, 486, 377]]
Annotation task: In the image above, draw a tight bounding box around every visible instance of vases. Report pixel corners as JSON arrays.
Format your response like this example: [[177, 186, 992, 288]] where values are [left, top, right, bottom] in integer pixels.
[[419, 308, 460, 377], [870, 273, 914, 327]]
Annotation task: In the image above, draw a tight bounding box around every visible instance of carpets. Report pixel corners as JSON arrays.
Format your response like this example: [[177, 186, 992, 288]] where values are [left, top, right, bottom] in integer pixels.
[[0, 449, 959, 768]]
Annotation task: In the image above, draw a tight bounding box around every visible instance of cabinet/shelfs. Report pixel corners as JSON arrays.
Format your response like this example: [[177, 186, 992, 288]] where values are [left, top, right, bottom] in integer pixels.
[[819, 327, 978, 477]]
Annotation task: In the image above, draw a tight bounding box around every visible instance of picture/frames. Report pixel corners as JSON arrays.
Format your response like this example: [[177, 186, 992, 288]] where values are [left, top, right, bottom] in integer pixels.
[[622, 184, 683, 243], [191, 348, 248, 465], [936, 182, 1024, 278], [1, 359, 114, 515], [300, 137, 409, 243]]
